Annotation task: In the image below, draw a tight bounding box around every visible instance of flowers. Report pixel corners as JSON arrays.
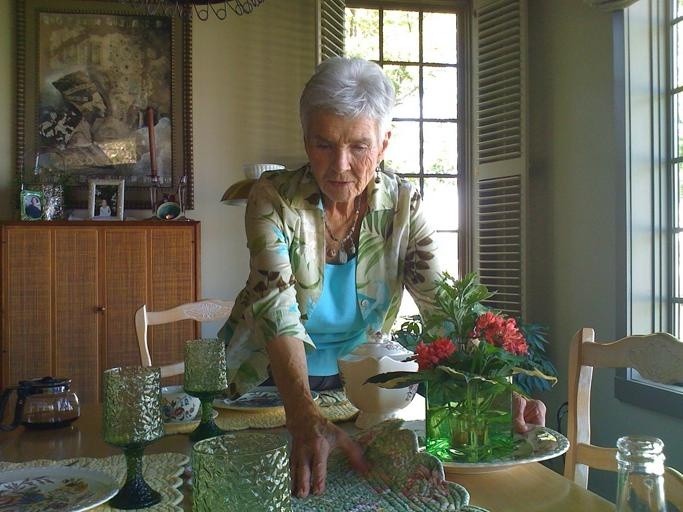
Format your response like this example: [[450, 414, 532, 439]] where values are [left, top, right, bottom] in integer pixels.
[[400, 270, 558, 465]]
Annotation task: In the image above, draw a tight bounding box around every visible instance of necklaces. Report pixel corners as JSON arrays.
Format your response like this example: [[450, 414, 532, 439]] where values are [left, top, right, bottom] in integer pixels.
[[323, 195, 360, 264]]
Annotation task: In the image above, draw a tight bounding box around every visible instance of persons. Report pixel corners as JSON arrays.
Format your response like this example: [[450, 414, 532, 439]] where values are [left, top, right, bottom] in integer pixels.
[[28, 197, 41, 218], [100, 199, 112, 216], [217, 56, 546, 499]]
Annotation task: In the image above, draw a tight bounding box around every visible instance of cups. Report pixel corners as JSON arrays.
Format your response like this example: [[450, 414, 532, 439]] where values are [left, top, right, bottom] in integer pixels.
[[40, 181, 64, 222], [187, 433, 292, 512]]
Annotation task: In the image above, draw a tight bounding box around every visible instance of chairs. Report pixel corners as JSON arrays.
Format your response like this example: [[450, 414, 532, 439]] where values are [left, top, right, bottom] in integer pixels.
[[564, 327, 683, 511], [135, 298, 237, 379]]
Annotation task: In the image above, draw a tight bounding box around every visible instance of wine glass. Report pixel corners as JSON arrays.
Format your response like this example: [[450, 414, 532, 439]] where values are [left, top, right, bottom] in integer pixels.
[[100, 363, 167, 509], [336, 331, 420, 431], [181, 338, 229, 439]]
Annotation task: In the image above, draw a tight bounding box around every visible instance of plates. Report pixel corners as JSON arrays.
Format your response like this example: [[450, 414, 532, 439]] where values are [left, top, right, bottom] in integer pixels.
[[162, 405, 219, 424], [211, 385, 319, 414], [397, 419, 571, 476], [0, 467, 120, 512]]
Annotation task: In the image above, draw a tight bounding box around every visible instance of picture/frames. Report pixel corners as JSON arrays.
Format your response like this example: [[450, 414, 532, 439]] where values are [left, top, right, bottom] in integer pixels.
[[20, 190, 44, 220], [14, 0, 194, 212], [88, 180, 124, 223]]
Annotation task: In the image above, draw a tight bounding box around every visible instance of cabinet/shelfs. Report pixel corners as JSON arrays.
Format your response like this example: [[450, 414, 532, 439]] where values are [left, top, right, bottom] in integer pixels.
[[1, 219, 201, 421]]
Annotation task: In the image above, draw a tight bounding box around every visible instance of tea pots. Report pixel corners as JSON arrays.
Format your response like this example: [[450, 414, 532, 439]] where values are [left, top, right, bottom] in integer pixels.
[[0, 376, 81, 433]]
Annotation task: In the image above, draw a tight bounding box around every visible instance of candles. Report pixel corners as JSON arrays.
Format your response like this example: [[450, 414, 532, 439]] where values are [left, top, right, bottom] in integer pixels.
[[146, 108, 156, 179]]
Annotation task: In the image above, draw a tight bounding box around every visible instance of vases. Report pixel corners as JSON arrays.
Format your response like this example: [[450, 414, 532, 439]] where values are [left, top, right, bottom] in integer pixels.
[[421, 375, 513, 464]]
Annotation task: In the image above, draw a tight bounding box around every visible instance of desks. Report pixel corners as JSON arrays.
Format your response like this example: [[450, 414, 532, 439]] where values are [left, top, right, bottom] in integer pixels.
[[0, 385, 618, 511]]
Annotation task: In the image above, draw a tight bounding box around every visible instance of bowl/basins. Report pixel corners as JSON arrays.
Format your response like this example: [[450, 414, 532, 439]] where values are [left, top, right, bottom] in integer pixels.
[[160, 384, 201, 422]]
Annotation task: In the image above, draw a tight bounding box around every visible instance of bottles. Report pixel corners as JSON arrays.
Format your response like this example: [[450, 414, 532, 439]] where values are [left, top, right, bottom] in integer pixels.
[[614, 433, 668, 512]]
